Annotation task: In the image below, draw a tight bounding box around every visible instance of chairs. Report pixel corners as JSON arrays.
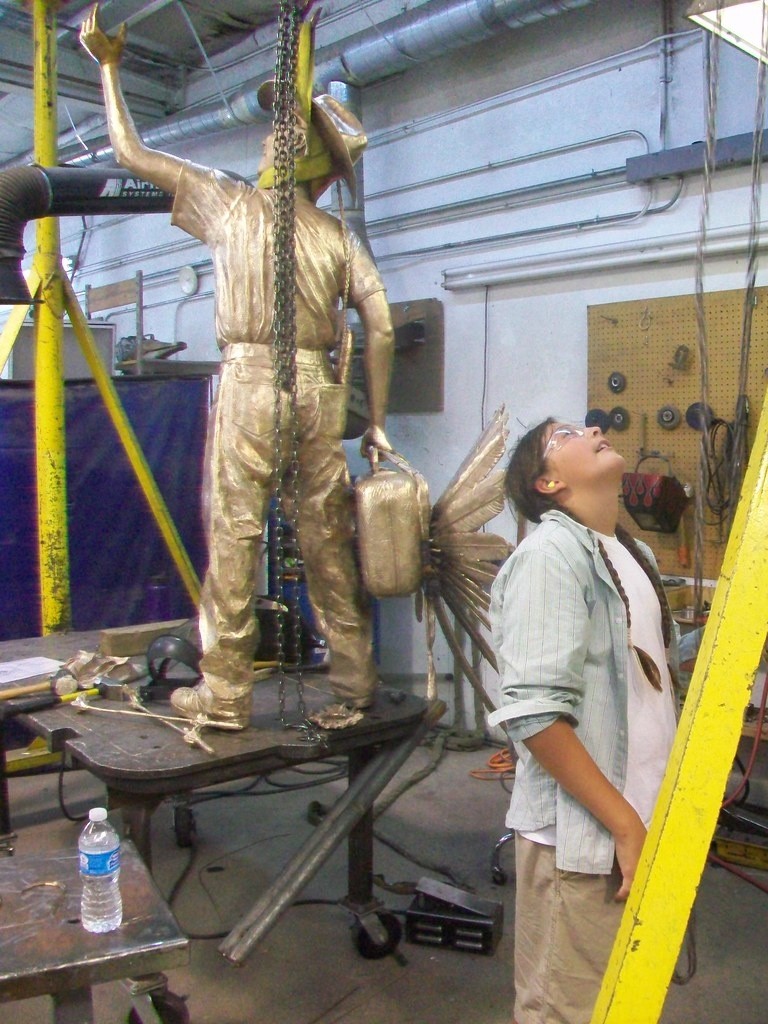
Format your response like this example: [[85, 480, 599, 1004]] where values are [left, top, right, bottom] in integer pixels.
[[85, 270, 220, 409]]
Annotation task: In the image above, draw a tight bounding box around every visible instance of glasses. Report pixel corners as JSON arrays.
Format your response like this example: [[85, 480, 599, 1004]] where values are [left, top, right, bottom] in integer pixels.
[[543, 427, 587, 460]]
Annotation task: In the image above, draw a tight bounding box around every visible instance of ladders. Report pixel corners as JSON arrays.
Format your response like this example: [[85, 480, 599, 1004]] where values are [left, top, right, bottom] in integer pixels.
[[593, 394, 768, 1024]]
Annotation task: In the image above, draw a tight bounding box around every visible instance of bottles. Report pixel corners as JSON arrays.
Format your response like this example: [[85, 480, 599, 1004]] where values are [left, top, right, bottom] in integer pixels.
[[144, 575, 172, 624], [77, 806, 123, 933]]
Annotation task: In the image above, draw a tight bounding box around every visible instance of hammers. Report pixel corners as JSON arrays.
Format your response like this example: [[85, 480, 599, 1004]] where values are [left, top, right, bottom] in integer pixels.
[[0, 668, 131, 717]]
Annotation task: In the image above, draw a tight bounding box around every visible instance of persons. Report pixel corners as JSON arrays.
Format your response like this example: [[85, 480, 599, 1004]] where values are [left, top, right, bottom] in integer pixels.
[[485, 408, 713, 1023], [78, 0, 399, 735]]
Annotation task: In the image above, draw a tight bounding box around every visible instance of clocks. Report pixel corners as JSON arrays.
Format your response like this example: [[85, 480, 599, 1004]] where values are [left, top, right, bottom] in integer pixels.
[[179, 266, 198, 295]]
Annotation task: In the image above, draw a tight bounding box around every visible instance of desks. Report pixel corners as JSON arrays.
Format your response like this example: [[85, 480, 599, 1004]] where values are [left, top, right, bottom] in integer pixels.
[[0, 839, 190, 1024], [0, 630, 430, 1024]]
